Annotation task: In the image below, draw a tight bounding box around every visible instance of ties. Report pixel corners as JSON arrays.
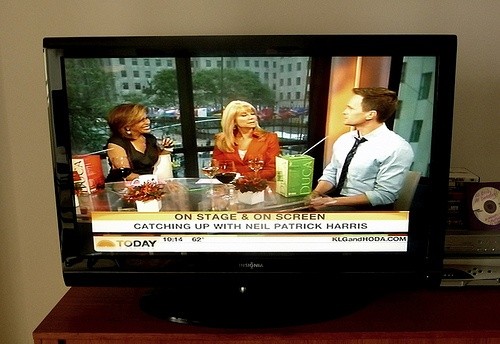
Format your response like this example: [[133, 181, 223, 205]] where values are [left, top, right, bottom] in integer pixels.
[[331, 137, 367, 196]]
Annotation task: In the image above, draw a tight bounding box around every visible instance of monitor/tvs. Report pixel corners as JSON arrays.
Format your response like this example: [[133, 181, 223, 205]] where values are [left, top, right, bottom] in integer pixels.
[[43, 35, 459, 327]]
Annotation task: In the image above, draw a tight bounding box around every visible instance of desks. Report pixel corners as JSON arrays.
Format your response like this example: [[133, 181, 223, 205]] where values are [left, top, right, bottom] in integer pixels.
[[33, 287, 500, 344], [73, 177, 343, 224]]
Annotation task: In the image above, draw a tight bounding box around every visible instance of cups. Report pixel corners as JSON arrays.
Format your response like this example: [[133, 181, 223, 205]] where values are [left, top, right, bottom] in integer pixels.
[[131, 174, 158, 187], [211, 184, 236, 210]]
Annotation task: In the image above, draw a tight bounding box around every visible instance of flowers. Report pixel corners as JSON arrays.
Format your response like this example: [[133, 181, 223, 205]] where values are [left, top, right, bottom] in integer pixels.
[[122, 181, 166, 204], [230, 175, 269, 193]]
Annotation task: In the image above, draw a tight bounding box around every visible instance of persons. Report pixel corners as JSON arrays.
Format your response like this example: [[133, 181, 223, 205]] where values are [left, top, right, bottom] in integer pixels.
[[306, 87, 414, 213], [105, 103, 173, 183], [212, 100, 280, 182]]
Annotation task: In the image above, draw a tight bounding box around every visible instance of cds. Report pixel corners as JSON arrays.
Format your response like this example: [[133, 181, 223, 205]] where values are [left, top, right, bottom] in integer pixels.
[[472, 187, 500, 226]]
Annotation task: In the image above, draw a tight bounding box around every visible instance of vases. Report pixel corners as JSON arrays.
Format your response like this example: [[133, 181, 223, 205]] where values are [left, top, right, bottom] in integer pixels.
[[134, 199, 163, 212], [237, 189, 264, 205]]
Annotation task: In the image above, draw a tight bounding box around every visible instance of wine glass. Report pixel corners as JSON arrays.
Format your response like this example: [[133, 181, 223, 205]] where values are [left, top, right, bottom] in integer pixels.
[[202, 158, 219, 196], [215, 160, 237, 200], [249, 154, 264, 178]]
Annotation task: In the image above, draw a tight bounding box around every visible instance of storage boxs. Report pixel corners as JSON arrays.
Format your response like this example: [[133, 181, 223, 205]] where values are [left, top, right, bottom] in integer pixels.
[[275, 154, 314, 199], [71, 154, 105, 194]]
